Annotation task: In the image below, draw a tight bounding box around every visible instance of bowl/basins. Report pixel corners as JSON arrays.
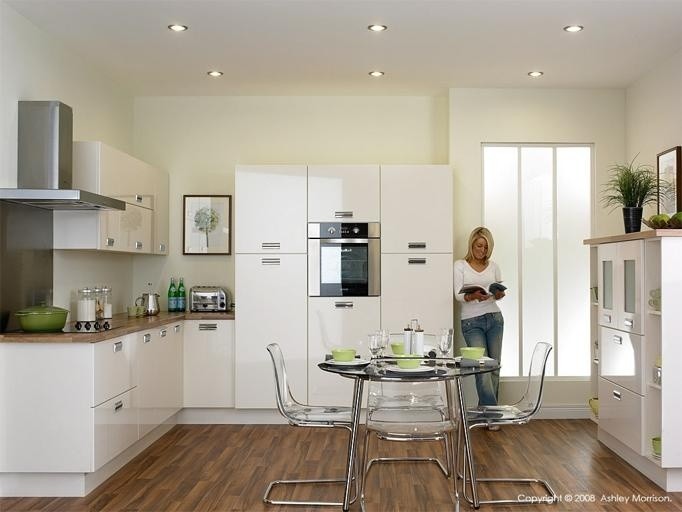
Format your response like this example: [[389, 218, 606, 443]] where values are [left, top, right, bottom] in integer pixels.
[[391, 343, 404, 354], [460, 347, 486, 358], [331, 349, 356, 361], [397, 359, 422, 368], [652, 437, 661, 455]]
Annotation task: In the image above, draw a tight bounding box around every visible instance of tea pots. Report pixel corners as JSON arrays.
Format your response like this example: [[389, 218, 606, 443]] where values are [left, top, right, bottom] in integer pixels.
[[135, 293, 161, 316]]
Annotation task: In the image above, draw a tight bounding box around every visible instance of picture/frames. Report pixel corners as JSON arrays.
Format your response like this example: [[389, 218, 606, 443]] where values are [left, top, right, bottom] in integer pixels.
[[183, 195, 231, 255], [656, 146, 682, 218]]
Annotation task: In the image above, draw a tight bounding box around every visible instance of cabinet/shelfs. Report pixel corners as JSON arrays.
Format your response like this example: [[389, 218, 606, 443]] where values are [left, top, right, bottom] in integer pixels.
[[235, 253, 307, 424], [154, 167, 169, 255], [135, 321, 183, 457], [379, 253, 453, 434], [0, 331, 136, 409], [73, 141, 153, 209], [598, 239, 645, 335], [309, 297, 380, 428], [1, 386, 144, 499], [182, 319, 235, 423], [381, 165, 455, 254], [53, 203, 153, 254], [596, 377, 643, 474], [235, 166, 307, 254], [308, 166, 381, 222], [590, 245, 598, 400], [643, 237, 681, 493], [598, 326, 648, 396]]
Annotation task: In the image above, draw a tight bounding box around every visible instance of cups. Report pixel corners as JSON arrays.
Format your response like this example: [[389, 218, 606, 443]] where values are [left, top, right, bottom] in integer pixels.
[[126, 307, 137, 318], [136, 306, 147, 317]]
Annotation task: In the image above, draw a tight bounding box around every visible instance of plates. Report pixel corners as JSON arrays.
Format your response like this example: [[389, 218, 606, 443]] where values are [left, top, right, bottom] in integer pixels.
[[386, 366, 434, 374], [454, 356, 495, 364], [325, 358, 370, 366]]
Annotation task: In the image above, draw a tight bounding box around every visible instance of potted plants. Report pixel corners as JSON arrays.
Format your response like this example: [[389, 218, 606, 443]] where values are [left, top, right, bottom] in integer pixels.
[[596, 152, 675, 234]]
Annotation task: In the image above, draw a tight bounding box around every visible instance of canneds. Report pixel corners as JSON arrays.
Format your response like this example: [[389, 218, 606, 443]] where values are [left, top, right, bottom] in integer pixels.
[[92, 289, 104, 319]]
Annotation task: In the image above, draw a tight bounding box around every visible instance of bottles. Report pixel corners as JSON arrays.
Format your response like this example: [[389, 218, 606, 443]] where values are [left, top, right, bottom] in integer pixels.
[[403, 324, 413, 354], [93, 287, 103, 321], [414, 325, 425, 357], [177, 277, 186, 312], [168, 277, 178, 312]]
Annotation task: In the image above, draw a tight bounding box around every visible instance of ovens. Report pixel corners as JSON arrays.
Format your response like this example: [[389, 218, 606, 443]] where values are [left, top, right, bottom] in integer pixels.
[[307, 222, 381, 297]]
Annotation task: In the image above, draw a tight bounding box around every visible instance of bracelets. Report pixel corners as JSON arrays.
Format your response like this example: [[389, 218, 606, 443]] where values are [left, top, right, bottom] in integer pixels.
[[467, 293, 472, 302]]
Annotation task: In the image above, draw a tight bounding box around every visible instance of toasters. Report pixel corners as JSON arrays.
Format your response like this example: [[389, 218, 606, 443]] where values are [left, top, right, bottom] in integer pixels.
[[189, 286, 232, 311]]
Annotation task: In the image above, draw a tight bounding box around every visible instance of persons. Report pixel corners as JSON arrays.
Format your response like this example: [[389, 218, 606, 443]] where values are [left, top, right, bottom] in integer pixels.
[[453, 227, 504, 431]]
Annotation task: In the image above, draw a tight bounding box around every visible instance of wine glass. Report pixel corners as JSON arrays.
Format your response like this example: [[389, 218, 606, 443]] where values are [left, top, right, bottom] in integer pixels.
[[367, 334, 382, 361], [438, 328, 453, 369], [374, 329, 390, 357]]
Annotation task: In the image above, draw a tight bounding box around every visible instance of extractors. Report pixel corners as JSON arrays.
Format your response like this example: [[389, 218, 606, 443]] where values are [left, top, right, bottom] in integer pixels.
[[1, 101, 125, 212]]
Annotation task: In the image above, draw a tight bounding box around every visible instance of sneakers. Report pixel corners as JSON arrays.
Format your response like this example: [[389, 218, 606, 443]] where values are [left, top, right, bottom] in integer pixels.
[[488, 419, 499, 431], [477, 418, 487, 430]]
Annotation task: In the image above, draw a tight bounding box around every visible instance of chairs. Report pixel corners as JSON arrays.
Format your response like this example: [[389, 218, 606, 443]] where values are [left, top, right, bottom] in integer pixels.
[[263, 343, 366, 504], [453, 342, 559, 505], [362, 333, 453, 479]]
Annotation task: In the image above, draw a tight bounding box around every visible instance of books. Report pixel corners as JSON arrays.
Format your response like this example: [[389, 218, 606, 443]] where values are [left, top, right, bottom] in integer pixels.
[[458, 281, 507, 302]]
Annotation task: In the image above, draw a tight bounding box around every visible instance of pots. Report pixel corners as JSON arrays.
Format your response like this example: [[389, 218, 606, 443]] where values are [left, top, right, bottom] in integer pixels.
[[14, 301, 70, 331]]
